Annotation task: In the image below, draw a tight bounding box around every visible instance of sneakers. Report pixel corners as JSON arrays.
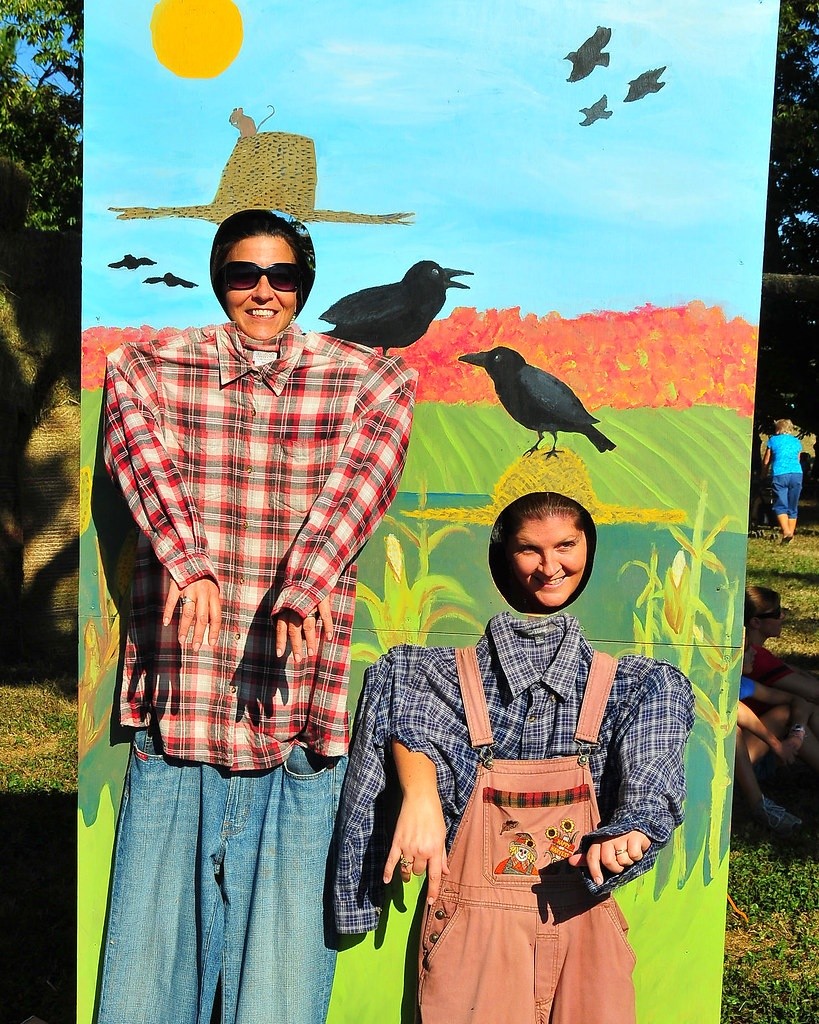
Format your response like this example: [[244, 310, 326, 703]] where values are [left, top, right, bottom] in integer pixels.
[[750, 793, 802, 838]]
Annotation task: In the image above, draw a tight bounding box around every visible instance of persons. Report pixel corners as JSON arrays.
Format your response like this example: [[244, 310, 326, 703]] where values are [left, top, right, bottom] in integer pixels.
[[733, 585, 819, 834], [105, 208, 422, 660], [381, 490, 698, 907], [762, 417, 805, 545]]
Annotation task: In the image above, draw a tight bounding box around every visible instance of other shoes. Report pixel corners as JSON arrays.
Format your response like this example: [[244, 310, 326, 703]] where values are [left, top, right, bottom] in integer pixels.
[[781, 536, 794, 545]]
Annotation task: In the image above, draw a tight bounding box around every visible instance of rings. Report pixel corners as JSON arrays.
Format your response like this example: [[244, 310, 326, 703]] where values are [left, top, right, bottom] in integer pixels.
[[400, 854, 416, 867], [615, 849, 628, 855], [182, 596, 196, 604]]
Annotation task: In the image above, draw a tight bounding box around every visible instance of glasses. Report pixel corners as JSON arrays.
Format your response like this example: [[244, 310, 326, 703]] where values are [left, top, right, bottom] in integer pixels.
[[755, 604, 781, 621], [218, 260, 302, 293]]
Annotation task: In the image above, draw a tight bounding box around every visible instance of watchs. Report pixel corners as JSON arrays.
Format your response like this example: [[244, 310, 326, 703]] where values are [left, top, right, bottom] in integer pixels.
[[791, 724, 809, 737]]
